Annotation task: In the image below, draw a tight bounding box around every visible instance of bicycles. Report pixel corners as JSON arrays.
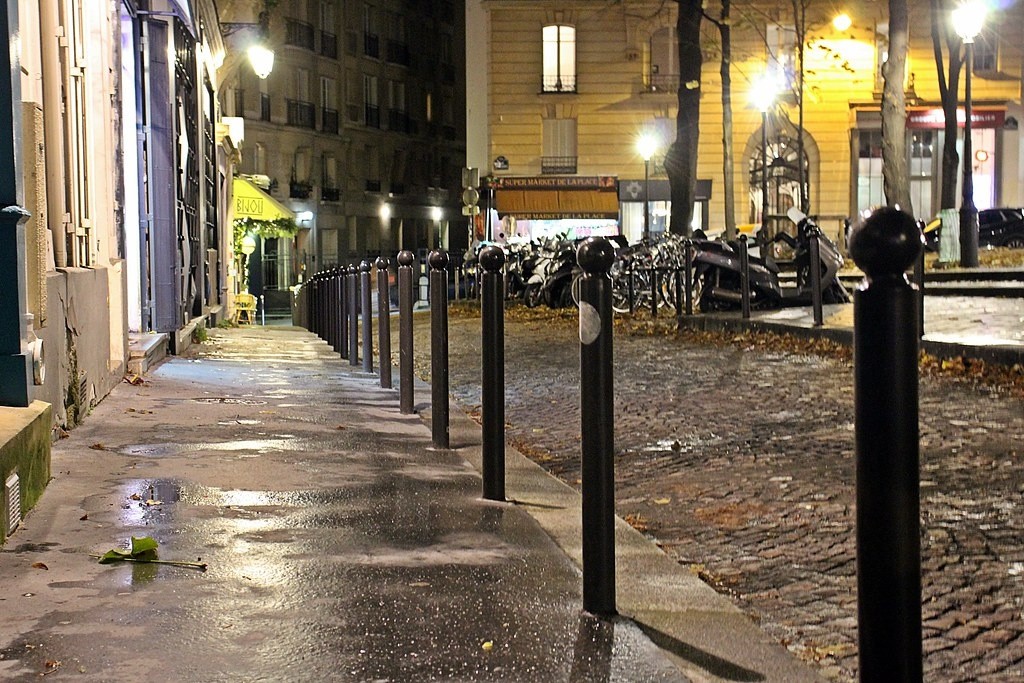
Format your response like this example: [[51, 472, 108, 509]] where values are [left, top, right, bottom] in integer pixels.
[[470, 232, 703, 313]]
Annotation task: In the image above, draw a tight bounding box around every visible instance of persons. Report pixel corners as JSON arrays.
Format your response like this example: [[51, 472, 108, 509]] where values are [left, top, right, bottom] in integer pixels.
[[299, 248, 307, 282]]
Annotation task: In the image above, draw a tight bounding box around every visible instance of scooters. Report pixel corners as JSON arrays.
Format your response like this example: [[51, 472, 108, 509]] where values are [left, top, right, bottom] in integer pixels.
[[691, 209, 851, 312]]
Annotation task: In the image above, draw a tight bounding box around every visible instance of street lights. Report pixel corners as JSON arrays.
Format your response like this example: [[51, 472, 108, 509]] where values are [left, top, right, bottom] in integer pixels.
[[637, 131, 657, 244], [950, 6, 987, 270], [750, 77, 781, 259]]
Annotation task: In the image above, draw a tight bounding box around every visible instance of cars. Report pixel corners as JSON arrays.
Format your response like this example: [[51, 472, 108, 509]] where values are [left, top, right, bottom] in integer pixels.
[[926, 207, 1024, 252]]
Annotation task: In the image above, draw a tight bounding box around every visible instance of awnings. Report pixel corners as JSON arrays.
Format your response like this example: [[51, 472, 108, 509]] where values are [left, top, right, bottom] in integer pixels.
[[496, 190, 619, 220], [233, 178, 294, 223]]
